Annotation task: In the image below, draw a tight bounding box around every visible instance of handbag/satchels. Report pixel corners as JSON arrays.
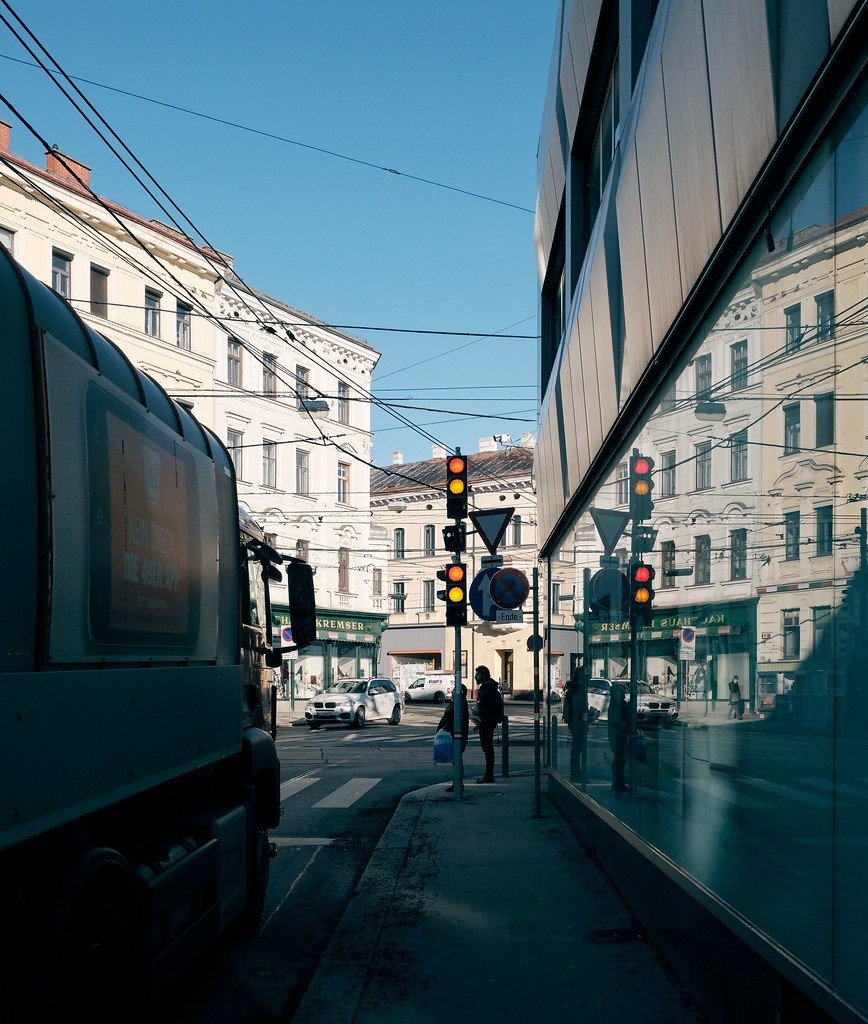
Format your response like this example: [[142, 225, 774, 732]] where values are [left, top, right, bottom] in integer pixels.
[[588, 706, 600, 724], [433, 729, 455, 762], [469, 704, 481, 724], [624, 735, 648, 763]]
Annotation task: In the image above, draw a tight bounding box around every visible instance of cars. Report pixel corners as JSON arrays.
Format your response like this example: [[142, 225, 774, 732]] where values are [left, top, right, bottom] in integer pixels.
[[562, 677, 680, 722], [404, 674, 455, 704], [304, 677, 404, 729]]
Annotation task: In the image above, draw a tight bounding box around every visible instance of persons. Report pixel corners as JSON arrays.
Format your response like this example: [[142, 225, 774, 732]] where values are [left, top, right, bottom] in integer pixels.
[[470, 665, 504, 784], [726, 676, 743, 720], [437, 684, 469, 792], [561, 666, 601, 783], [608, 684, 647, 791]]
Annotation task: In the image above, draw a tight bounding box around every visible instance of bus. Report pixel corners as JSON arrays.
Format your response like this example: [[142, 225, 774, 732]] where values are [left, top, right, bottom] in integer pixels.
[[1, 240, 321, 1022]]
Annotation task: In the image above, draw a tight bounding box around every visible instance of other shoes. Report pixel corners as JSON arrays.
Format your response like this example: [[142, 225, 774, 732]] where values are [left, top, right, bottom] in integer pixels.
[[570, 779, 590, 785], [477, 777, 495, 783], [445, 784, 465, 792], [613, 785, 630, 792]]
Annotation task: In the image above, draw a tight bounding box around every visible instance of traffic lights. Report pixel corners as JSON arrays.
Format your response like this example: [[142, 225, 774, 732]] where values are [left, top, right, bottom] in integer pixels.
[[446, 455, 468, 519], [630, 562, 655, 628], [446, 563, 467, 625], [629, 456, 655, 519]]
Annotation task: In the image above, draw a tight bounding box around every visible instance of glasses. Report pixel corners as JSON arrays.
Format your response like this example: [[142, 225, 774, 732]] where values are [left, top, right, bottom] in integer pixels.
[[476, 673, 480, 676]]
